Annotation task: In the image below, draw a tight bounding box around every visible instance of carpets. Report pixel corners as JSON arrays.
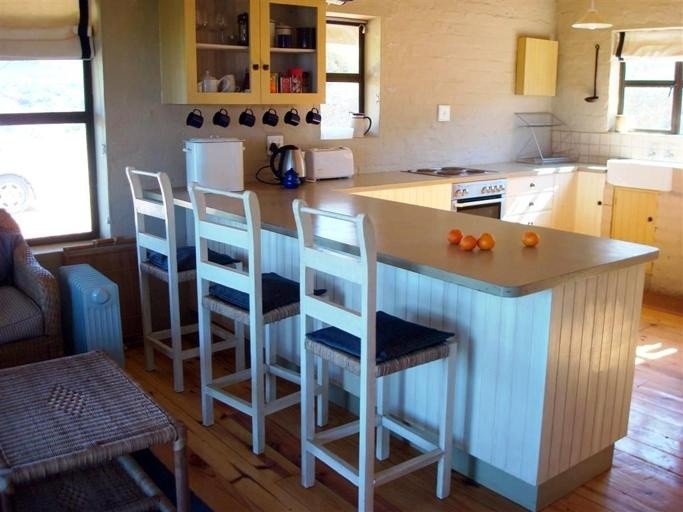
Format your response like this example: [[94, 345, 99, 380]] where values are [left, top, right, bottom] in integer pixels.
[[7, 445, 214, 512]]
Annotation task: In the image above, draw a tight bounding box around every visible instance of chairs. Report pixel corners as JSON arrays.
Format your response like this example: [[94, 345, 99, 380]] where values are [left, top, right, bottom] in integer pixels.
[[0, 209, 65, 372]]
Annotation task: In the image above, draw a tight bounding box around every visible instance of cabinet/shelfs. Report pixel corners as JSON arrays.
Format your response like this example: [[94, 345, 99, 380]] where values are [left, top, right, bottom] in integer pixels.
[[514, 36, 559, 99], [158, 0, 327, 107], [508, 174, 577, 232], [577, 172, 605, 239], [610, 188, 658, 277]]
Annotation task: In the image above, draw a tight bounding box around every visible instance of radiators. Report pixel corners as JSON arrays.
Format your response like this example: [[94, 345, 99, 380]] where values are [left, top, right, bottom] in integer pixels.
[[58, 264, 122, 365]]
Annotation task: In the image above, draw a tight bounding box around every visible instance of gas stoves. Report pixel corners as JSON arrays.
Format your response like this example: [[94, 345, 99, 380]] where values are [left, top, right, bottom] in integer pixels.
[[400, 166, 499, 178]]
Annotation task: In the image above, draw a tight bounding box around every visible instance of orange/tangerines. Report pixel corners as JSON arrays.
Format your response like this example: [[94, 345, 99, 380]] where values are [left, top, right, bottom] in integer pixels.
[[521, 232, 538, 246], [460, 236, 478, 250], [447, 229, 464, 244], [478, 233, 495, 250]]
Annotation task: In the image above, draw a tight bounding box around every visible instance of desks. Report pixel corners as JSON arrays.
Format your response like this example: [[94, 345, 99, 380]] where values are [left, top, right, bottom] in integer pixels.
[[2, 349, 189, 511]]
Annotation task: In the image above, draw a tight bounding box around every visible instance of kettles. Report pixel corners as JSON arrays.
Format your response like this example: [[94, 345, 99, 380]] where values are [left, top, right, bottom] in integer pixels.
[[269, 144, 307, 182]]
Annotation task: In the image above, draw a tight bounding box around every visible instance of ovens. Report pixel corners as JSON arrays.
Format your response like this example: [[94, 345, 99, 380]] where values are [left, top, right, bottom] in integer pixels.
[[451, 179, 507, 222]]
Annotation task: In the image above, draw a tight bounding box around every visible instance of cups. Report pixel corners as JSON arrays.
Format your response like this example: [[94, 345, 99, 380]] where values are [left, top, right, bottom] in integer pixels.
[[213, 109, 231, 128], [238, 108, 256, 127], [307, 108, 321, 124], [262, 108, 279, 126], [350, 112, 371, 138], [186, 109, 205, 128], [284, 108, 300, 126]]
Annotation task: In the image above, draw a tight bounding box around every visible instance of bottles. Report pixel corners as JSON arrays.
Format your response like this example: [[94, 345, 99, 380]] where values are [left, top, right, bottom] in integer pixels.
[[297, 27, 315, 50], [276, 24, 296, 48], [238, 12, 250, 45], [288, 67, 303, 93], [223, 74, 236, 92]]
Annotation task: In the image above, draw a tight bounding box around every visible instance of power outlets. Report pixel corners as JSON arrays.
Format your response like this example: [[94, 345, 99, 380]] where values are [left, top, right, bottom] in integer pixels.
[[265, 135, 284, 157]]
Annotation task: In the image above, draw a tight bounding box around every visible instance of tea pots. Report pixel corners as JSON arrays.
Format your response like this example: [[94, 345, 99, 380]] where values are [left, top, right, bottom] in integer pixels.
[[200, 71, 230, 92]]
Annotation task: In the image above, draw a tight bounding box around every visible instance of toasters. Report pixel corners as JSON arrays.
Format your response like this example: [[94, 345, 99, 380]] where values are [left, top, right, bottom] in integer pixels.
[[304, 146, 354, 181]]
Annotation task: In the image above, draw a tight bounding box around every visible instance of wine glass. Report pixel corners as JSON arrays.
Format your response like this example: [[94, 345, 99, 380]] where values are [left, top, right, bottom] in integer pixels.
[[215, 13, 228, 43], [198, 10, 208, 42]]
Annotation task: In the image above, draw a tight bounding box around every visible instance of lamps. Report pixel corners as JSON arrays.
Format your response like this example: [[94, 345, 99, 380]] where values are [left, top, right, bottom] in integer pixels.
[[570, 0, 614, 31]]
